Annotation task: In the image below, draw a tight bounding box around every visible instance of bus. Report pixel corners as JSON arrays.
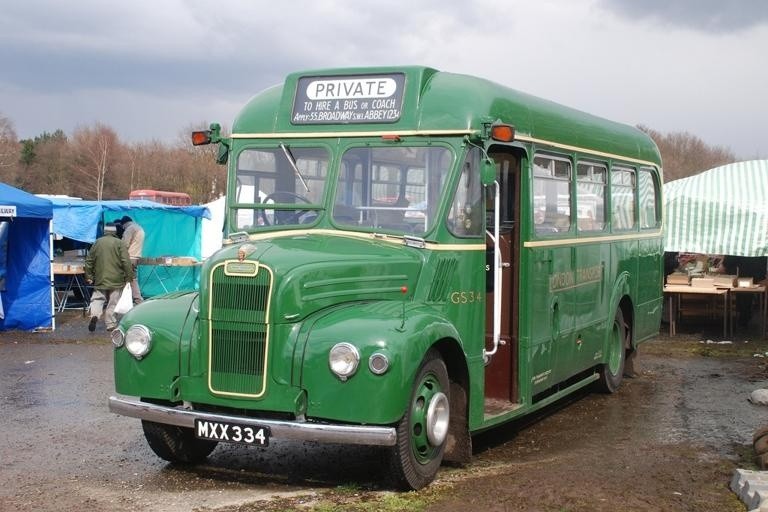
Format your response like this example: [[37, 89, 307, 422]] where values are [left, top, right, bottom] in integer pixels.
[[109, 67, 666, 489], [128, 190, 192, 206]]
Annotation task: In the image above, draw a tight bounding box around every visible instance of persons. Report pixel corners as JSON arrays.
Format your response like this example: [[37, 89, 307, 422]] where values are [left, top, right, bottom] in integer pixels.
[[717, 256, 767, 328], [119, 215, 143, 303], [85, 222, 137, 332], [664, 252, 681, 284]]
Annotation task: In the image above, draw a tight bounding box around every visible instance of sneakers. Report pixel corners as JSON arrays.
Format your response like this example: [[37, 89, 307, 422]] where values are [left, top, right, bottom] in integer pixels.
[[88, 316, 98, 332], [106, 322, 118, 330]]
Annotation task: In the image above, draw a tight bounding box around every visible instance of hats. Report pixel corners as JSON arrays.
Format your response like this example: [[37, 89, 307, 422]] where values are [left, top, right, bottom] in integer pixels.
[[103, 222, 117, 231], [121, 215, 131, 225]]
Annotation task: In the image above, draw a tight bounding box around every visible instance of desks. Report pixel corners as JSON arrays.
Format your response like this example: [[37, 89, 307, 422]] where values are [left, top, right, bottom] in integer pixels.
[[662, 284, 768, 340], [137, 260, 204, 296], [53, 269, 93, 313]]
